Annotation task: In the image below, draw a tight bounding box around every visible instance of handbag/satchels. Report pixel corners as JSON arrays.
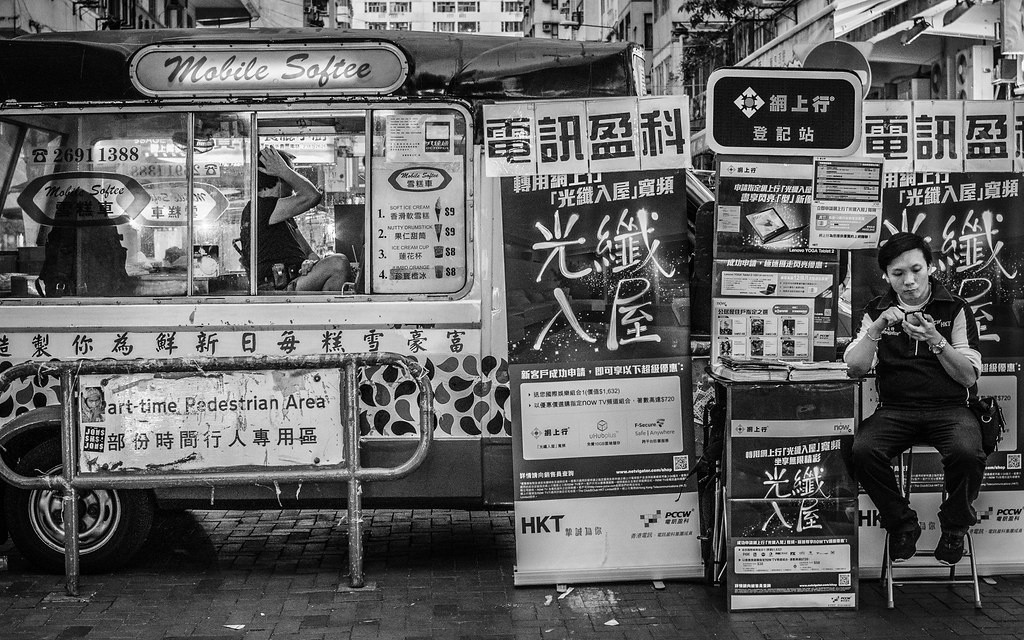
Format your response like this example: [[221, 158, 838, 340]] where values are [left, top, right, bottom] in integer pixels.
[[969, 396, 1006, 456]]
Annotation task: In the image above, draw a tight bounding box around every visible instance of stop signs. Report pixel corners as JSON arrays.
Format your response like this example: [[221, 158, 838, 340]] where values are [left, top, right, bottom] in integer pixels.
[[805, 43, 870, 101]]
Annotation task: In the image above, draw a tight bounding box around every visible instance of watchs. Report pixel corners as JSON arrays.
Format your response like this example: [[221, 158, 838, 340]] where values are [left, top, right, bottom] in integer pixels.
[[927, 338, 946, 354]]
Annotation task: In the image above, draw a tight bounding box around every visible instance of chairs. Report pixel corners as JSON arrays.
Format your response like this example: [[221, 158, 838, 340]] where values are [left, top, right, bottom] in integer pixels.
[[232, 238, 279, 291], [876, 379, 982, 611]]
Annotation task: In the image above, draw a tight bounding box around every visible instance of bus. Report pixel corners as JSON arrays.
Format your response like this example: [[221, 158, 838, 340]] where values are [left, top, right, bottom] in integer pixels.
[[0, 28, 717, 578]]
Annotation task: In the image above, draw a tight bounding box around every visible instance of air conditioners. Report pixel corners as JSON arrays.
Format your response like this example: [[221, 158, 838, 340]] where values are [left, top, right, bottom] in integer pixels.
[[526, 9, 529, 13], [931, 57, 957, 100], [898, 78, 932, 100], [544, 24, 550, 29], [956, 46, 994, 101], [691, 90, 707, 120]]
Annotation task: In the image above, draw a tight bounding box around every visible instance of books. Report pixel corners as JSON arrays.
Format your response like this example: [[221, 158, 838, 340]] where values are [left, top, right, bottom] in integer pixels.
[[715, 353, 849, 380]]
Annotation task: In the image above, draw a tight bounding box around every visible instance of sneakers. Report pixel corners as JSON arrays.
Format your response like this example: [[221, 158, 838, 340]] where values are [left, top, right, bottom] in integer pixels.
[[934, 532, 964, 566], [889, 525, 922, 562]]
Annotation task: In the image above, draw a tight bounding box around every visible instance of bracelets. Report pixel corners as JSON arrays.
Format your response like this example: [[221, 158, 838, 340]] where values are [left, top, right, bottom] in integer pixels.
[[867, 326, 883, 341]]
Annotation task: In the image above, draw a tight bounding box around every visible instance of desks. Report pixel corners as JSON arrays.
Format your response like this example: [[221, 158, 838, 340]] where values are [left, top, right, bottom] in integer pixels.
[[131, 275, 218, 295]]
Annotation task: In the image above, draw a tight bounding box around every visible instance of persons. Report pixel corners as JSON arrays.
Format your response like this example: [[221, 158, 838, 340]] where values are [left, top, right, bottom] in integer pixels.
[[843, 233, 988, 567], [240, 145, 351, 292]]
